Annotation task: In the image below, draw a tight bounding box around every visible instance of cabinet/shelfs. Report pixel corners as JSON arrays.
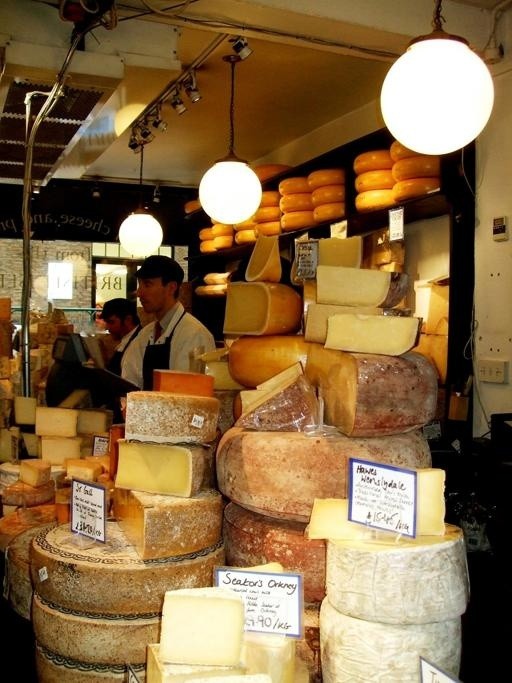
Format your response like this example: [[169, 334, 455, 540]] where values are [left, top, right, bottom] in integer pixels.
[[182, 122, 476, 259]]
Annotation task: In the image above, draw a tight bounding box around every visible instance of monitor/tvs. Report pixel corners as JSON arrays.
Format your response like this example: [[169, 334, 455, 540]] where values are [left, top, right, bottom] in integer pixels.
[[52, 332, 95, 365]]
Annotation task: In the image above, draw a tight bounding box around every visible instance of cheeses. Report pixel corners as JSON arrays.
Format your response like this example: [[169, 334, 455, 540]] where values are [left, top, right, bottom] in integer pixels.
[[0, 139, 470, 683]]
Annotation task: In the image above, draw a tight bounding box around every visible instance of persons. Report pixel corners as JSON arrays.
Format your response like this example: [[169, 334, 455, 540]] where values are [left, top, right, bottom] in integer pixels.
[[99, 298, 143, 423], [120, 255, 216, 420]]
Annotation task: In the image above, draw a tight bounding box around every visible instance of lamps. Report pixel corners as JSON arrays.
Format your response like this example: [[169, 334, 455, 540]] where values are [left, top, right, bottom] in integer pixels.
[[200, 56, 264, 226], [378, 0, 494, 156], [127, 35, 253, 155], [119, 144, 165, 257]]
